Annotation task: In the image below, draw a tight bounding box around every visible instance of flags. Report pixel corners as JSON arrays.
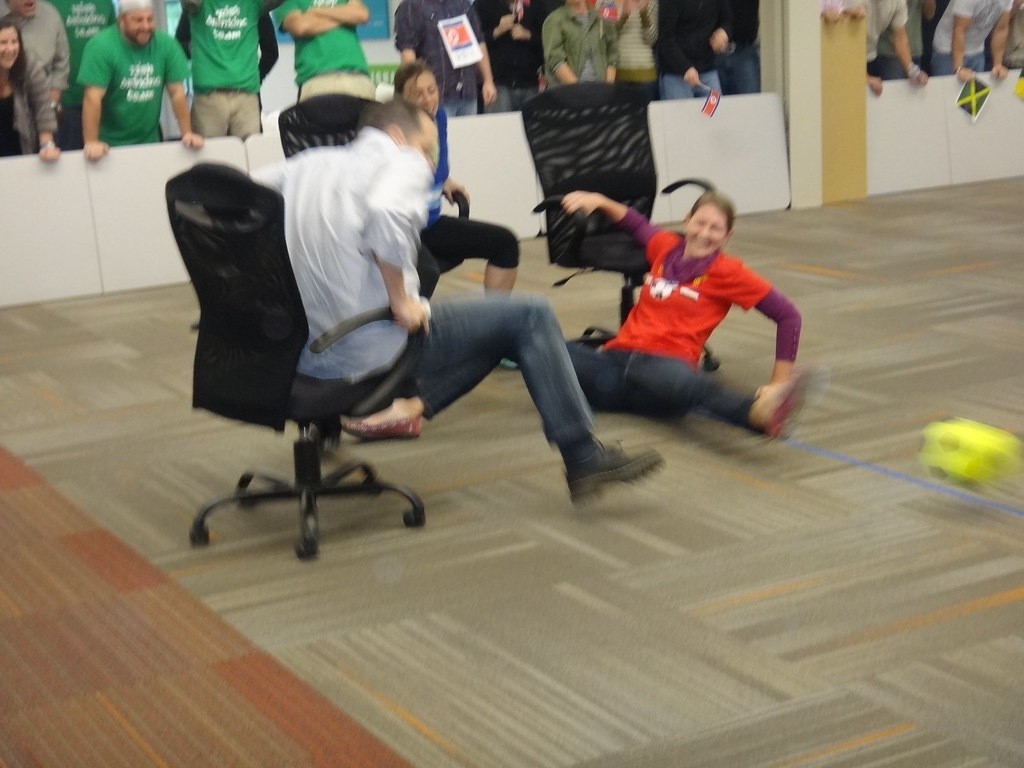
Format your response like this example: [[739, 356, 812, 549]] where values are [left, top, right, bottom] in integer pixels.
[[597, 3, 618, 23], [514, 1, 524, 22]]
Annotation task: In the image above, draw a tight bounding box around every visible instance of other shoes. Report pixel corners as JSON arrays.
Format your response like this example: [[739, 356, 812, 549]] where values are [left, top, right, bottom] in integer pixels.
[[563, 441, 663, 505], [753, 372, 811, 438], [340, 414, 421, 439]]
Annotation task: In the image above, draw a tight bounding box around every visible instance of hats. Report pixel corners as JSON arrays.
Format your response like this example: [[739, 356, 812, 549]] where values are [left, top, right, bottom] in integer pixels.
[[116, 0, 154, 19]]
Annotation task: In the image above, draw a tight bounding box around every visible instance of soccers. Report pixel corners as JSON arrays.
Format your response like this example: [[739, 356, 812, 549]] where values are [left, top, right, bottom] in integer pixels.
[[920, 418, 1017, 489]]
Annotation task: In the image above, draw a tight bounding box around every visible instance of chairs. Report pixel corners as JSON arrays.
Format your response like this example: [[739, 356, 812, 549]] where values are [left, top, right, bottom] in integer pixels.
[[521, 79, 721, 373], [163, 162, 427, 562], [278, 91, 471, 272]]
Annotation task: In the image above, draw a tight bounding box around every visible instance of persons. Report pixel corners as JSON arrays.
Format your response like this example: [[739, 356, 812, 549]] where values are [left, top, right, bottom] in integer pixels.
[[473, 0, 546, 112], [3, 1, 73, 112], [394, 0, 498, 116], [0, 17, 63, 164], [392, 60, 522, 372], [340, 190, 810, 442], [656, 0, 735, 97], [602, 0, 660, 97], [272, 0, 378, 104], [714, 0, 1024, 96], [247, 98, 666, 503], [76, 0, 205, 163], [540, 0, 619, 89], [174, 0, 287, 140]]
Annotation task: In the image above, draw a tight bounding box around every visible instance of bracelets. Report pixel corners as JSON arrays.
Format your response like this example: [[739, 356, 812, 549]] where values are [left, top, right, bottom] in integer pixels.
[[50, 100, 63, 112], [954, 64, 963, 74], [39, 140, 56, 146]]
